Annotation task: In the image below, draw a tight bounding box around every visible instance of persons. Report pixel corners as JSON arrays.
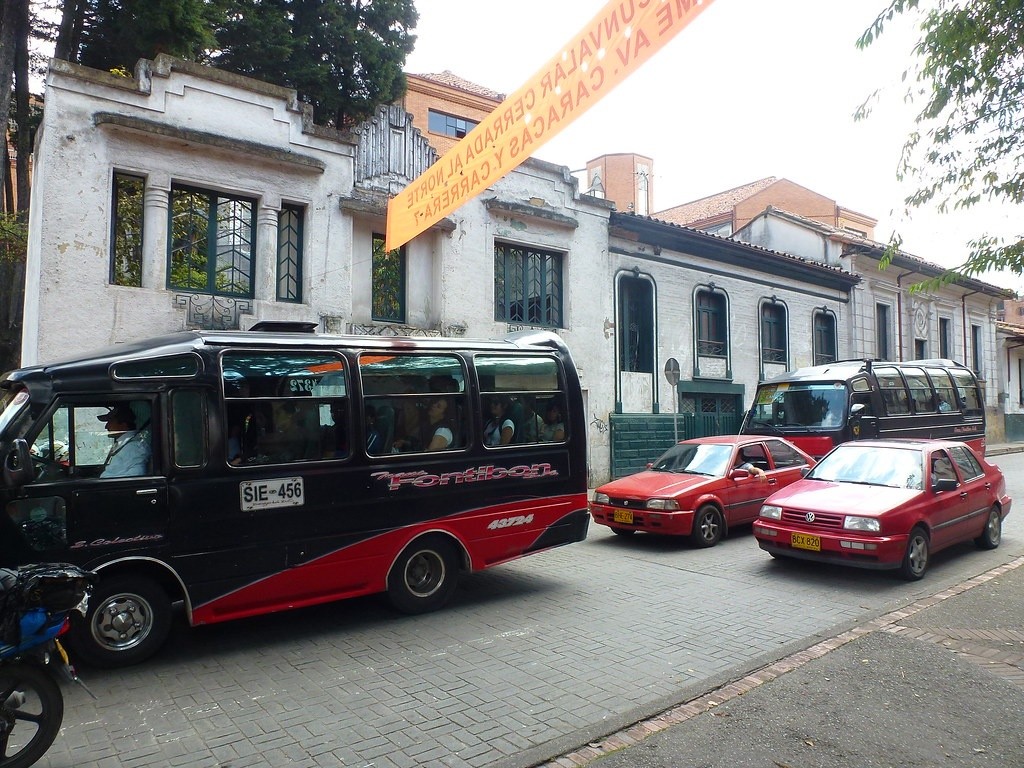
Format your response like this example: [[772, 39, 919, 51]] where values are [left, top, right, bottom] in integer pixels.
[[319, 398, 380, 459], [392, 380, 566, 451], [31, 440, 70, 466], [228, 398, 306, 465], [939, 392, 951, 411], [97, 407, 150, 478], [733, 448, 767, 483]]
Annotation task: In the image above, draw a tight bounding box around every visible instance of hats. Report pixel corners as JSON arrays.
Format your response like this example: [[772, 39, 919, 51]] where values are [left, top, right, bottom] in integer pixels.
[[96, 406, 136, 422]]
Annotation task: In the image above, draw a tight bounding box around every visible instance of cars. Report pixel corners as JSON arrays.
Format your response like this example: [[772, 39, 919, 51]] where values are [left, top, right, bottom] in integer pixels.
[[753, 435, 1013, 582], [590, 433, 817, 548]]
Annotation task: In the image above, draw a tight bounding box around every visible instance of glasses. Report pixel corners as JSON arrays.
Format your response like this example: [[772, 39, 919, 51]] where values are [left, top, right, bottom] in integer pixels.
[[488, 399, 503, 405]]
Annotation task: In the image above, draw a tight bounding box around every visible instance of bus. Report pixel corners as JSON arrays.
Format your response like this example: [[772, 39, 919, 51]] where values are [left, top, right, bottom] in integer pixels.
[[0, 318, 591, 666], [738, 357, 987, 466]]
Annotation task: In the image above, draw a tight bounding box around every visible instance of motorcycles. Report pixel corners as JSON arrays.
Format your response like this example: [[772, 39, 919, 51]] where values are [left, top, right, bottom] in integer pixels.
[[1, 563, 102, 768]]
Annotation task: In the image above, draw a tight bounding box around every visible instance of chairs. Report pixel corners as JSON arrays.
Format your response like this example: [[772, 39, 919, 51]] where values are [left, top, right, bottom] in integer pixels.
[[130, 400, 153, 474], [452, 404, 464, 448], [302, 406, 322, 459], [750, 462, 768, 471], [377, 406, 395, 454], [508, 402, 525, 443]]
